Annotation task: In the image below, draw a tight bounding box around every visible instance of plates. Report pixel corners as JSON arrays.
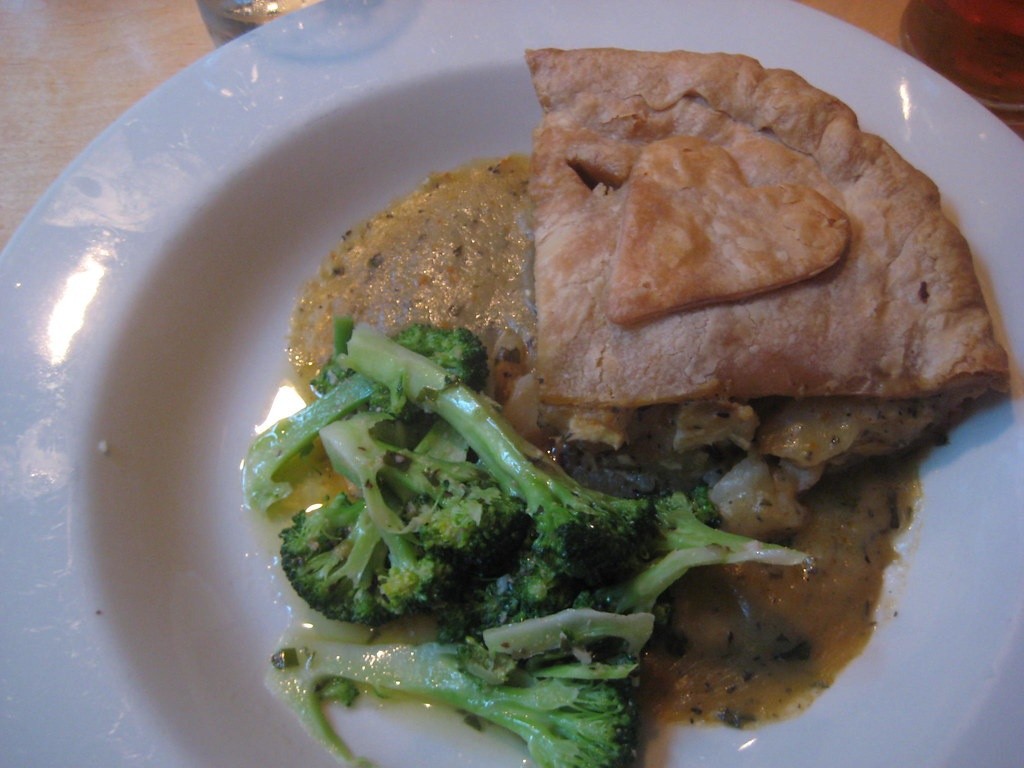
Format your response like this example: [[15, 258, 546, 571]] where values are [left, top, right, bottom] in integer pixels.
[[0, 0, 1024, 768]]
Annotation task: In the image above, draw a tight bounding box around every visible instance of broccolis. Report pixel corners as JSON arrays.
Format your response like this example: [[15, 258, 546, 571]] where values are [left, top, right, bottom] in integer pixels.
[[240, 317, 808, 768]]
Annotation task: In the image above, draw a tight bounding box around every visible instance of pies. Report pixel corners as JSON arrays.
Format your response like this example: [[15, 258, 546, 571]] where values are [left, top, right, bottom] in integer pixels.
[[528, 45, 1011, 406]]
